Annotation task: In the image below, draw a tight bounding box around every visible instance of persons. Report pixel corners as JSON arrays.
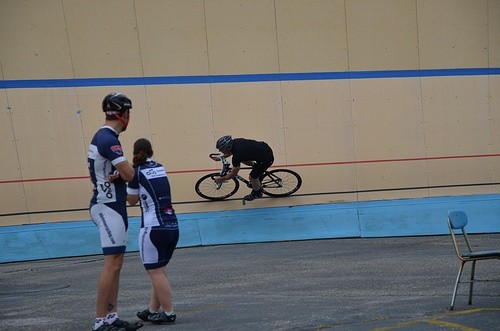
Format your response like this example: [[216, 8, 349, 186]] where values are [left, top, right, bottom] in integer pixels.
[[127, 139, 179, 324], [88, 93, 143, 331], [216, 136, 274, 201]]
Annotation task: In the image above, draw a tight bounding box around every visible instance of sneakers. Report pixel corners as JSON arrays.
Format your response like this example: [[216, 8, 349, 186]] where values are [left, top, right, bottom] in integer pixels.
[[147, 312, 176, 325], [136, 309, 160, 321], [92, 322, 125, 331], [110, 318, 144, 330], [244, 188, 263, 201]]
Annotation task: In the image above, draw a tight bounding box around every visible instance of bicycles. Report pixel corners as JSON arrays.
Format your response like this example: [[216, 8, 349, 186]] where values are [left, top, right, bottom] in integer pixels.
[[195, 153, 302, 200]]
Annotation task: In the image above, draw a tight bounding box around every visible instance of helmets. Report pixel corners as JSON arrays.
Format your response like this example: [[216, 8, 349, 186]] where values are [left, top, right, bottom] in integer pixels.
[[102, 92, 132, 112], [216, 135, 233, 150]]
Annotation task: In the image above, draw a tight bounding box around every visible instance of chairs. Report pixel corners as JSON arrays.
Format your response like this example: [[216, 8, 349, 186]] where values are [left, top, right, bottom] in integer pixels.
[[447, 209, 500, 311]]
